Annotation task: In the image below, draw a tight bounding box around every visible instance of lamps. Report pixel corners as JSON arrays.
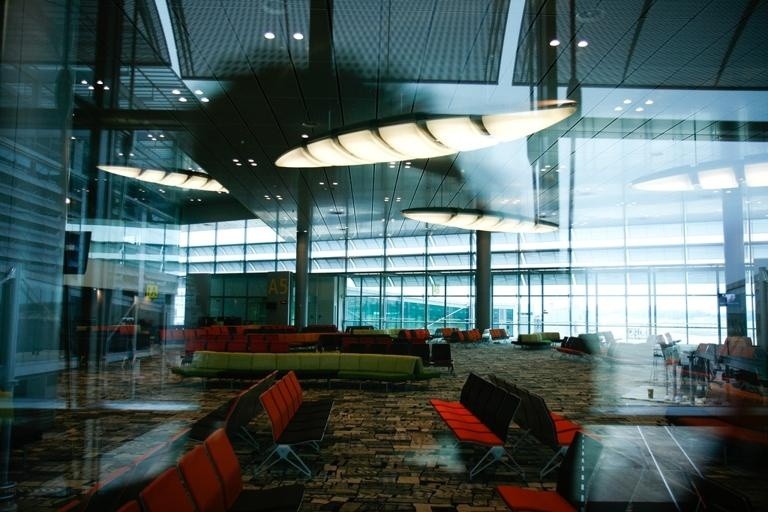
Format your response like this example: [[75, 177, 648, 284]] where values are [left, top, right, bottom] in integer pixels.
[[273, 100, 579, 169], [628, 151, 767, 193], [95, 163, 230, 194], [401, 204, 561, 234]]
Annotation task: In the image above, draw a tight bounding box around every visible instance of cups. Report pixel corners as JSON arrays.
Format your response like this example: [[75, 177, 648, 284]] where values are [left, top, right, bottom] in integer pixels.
[[647, 388, 654, 399]]
